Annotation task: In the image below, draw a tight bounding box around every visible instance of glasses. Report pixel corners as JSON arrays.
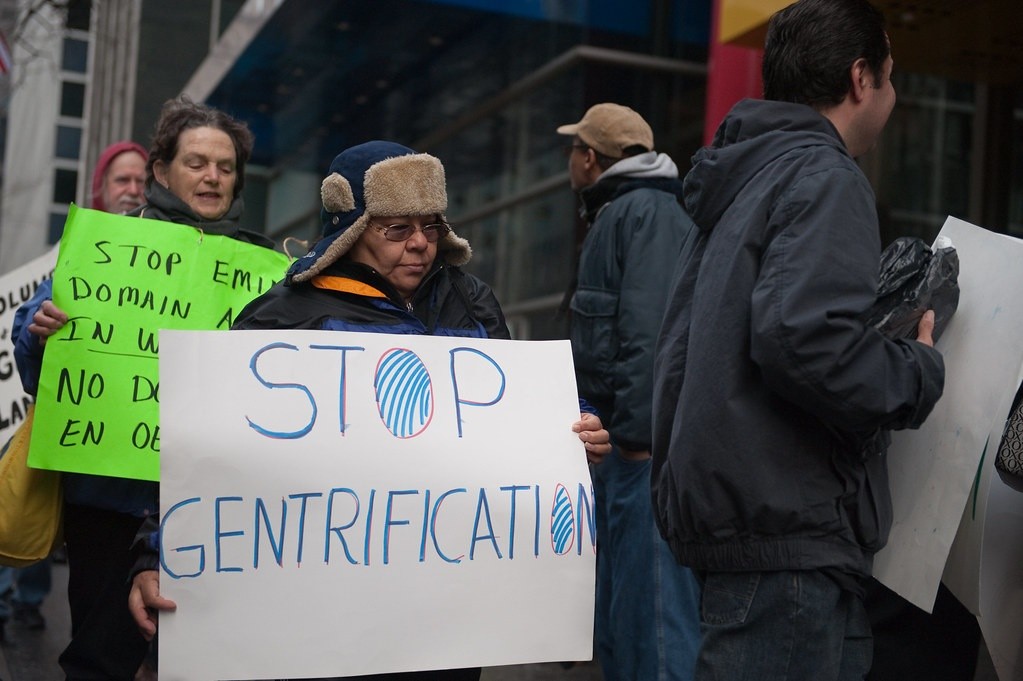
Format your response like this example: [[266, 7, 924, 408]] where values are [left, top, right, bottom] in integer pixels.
[[367, 218, 450, 243]]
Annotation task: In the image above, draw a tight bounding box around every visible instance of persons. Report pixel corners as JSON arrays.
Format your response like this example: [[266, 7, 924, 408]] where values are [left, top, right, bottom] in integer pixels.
[[557, 102, 701, 681], [650, 0, 946, 681], [2, 96, 274, 680], [118, 141, 610, 681]]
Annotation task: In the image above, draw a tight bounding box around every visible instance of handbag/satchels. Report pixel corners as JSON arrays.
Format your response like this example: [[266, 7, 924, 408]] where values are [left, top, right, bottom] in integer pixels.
[[0, 402, 66, 567]]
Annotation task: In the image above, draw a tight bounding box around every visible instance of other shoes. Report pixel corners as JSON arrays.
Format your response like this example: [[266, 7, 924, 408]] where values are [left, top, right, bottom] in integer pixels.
[[13, 605, 44, 630]]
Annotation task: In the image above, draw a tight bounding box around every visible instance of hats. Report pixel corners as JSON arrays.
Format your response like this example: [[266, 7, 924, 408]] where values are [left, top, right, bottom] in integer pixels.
[[284, 141, 469, 288], [556, 103, 653, 157]]
